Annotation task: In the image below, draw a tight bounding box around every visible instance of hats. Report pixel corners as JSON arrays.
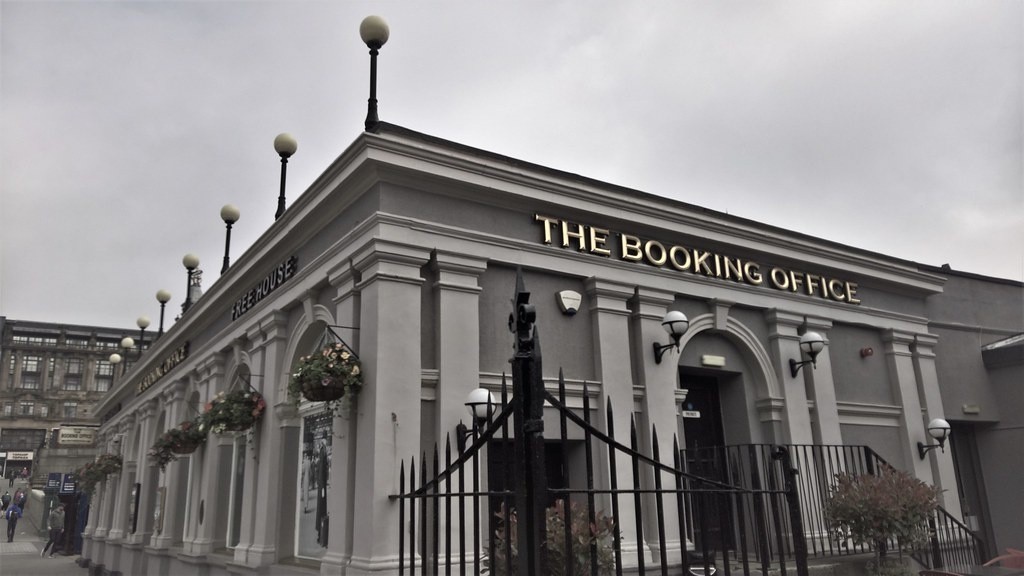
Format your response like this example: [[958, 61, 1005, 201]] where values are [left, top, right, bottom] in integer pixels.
[[58, 503, 65, 508]]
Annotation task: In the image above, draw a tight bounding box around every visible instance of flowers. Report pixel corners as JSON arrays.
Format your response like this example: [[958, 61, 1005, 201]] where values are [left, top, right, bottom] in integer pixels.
[[69, 453, 124, 505], [278, 340, 368, 439], [196, 389, 267, 466], [147, 420, 207, 473]]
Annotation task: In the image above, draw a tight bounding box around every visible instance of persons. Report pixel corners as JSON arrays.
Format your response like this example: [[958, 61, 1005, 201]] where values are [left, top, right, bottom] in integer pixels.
[[8, 468, 15, 487], [6, 500, 23, 542], [1, 491, 11, 512], [21, 467, 28, 480], [0, 498, 3, 518], [13, 487, 27, 517], [41, 503, 65, 559]]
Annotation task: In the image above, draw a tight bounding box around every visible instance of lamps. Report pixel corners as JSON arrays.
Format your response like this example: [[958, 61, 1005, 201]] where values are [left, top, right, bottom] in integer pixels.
[[360, 15, 391, 132], [220, 203, 240, 276], [109, 353, 122, 388], [273, 133, 298, 222], [155, 288, 171, 341], [653, 311, 690, 365], [121, 337, 135, 376], [181, 252, 200, 315], [456, 387, 498, 452], [136, 316, 151, 359], [917, 419, 951, 461], [789, 332, 824, 379]]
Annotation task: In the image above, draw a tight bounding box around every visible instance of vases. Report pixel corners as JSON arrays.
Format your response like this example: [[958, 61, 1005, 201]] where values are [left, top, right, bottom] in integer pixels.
[[100, 466, 115, 474], [302, 378, 345, 401], [223, 417, 261, 431], [170, 441, 199, 454]]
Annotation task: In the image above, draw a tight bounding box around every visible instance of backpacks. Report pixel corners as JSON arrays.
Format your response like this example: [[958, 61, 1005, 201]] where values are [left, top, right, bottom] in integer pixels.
[[9, 507, 19, 523]]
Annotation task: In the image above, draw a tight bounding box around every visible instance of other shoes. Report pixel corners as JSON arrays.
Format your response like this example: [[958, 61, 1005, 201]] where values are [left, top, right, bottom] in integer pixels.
[[41, 548, 46, 558], [48, 554, 56, 559]]
